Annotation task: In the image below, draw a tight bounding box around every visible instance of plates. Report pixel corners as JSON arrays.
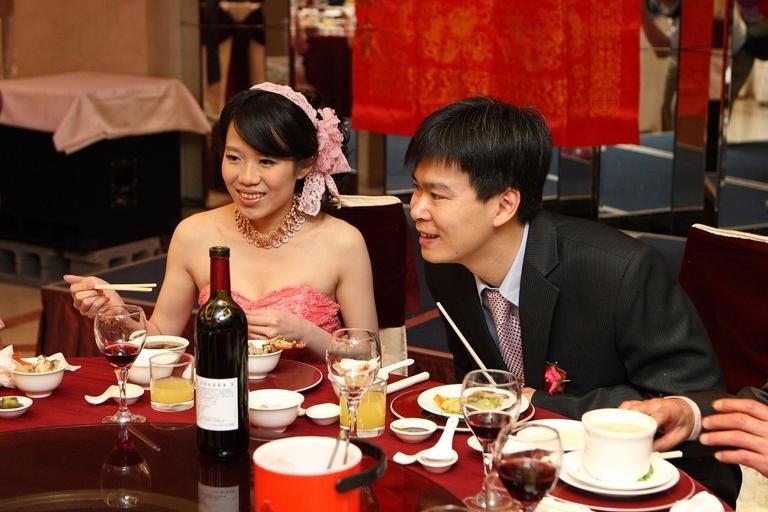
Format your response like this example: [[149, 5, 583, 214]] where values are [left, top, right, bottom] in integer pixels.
[[514, 419, 583, 451], [415, 383, 530, 421], [565, 450, 674, 491], [0, 395, 34, 419], [461, 387, 516, 412], [557, 449, 680, 498], [389, 417, 437, 443], [83, 382, 145, 406], [465, 433, 520, 459], [391, 447, 458, 474]]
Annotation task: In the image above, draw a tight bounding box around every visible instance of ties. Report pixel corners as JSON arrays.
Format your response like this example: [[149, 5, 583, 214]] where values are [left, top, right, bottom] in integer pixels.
[[486, 291, 525, 387]]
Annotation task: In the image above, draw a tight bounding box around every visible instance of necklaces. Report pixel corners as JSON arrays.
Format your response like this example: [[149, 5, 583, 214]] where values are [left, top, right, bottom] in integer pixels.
[[231, 194, 307, 250]]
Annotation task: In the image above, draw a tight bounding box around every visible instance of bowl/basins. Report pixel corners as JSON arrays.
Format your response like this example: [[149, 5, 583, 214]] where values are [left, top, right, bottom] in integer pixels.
[[247, 388, 304, 433], [117, 336, 189, 384], [247, 340, 283, 380], [6, 356, 66, 399], [304, 403, 340, 426]]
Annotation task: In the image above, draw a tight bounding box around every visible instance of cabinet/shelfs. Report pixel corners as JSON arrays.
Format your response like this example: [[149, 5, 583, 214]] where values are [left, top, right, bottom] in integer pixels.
[[0, 71, 185, 254]]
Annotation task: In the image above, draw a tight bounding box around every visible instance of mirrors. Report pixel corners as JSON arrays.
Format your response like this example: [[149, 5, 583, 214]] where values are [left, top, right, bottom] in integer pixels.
[[199, 0, 267, 208], [595, 1, 709, 219], [710, 1, 767, 231], [289, 1, 359, 195]]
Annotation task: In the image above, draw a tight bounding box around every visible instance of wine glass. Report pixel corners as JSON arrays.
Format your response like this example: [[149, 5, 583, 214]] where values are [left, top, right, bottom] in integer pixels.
[[325, 328, 383, 441], [461, 370, 522, 509], [93, 303, 148, 424], [493, 423, 563, 512], [99, 427, 153, 510]]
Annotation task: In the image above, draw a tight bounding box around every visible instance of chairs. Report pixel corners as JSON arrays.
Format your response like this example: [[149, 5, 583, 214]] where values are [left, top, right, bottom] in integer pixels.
[[201, 2, 268, 118], [323, 194, 410, 377], [678, 222, 768, 396]]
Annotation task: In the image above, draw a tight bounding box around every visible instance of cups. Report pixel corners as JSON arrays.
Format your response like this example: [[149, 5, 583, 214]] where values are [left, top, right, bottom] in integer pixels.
[[579, 408, 658, 483], [481, 469, 522, 512], [148, 351, 195, 413]]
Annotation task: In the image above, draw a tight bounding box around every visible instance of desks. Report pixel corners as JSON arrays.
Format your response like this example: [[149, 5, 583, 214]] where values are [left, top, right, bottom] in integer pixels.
[[35, 254, 200, 357]]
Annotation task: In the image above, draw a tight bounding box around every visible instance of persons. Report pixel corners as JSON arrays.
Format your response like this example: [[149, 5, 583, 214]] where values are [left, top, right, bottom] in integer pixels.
[[401, 93, 741, 511], [61, 82, 379, 362], [617, 380, 768, 487]]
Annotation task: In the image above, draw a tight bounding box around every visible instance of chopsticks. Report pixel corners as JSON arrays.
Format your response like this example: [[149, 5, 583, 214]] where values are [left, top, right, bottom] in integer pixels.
[[94, 282, 157, 294], [434, 300, 504, 387]]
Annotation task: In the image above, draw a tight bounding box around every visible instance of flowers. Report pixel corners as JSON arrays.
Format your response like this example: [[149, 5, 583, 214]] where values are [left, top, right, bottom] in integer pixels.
[[313, 106, 347, 177], [541, 362, 569, 394]]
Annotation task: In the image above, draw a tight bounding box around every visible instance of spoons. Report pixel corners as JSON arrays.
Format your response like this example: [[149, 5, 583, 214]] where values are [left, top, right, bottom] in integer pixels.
[[420, 416, 459, 462]]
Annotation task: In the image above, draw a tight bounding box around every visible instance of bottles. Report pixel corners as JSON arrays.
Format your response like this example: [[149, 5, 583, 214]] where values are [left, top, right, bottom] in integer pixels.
[[196, 462, 250, 512], [194, 245, 249, 463]]
